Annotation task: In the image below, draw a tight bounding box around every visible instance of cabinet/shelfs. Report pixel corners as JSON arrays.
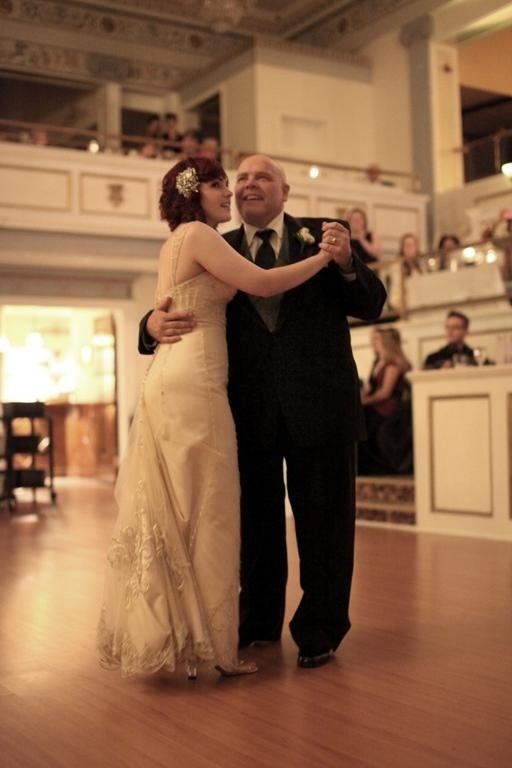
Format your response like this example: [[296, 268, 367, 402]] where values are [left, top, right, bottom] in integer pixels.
[[0, 401, 57, 509]]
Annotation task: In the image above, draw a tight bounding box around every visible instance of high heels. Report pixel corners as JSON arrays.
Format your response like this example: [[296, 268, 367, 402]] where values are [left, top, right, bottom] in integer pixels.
[[186, 659, 259, 680]]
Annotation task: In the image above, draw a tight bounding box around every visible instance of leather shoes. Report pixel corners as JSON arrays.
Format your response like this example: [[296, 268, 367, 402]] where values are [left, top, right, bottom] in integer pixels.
[[296, 650, 330, 668]]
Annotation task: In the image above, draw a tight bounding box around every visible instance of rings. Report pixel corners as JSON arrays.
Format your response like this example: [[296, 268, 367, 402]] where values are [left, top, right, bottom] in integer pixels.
[[331, 235, 338, 244]]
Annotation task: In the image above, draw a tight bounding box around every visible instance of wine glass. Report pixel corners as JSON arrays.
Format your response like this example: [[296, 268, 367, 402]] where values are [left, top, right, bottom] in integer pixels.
[[473, 347, 487, 366]]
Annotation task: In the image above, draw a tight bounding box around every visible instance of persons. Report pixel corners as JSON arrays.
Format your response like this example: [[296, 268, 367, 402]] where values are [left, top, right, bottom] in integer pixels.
[[436, 235, 474, 270], [397, 233, 431, 276], [94, 155, 335, 686], [368, 329, 389, 394], [421, 309, 481, 368], [346, 204, 383, 264], [1, 111, 220, 160], [140, 157, 386, 668], [361, 326, 412, 472]]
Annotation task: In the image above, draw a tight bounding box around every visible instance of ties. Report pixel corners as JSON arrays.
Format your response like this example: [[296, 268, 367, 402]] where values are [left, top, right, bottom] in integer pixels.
[[254, 229, 277, 268]]
